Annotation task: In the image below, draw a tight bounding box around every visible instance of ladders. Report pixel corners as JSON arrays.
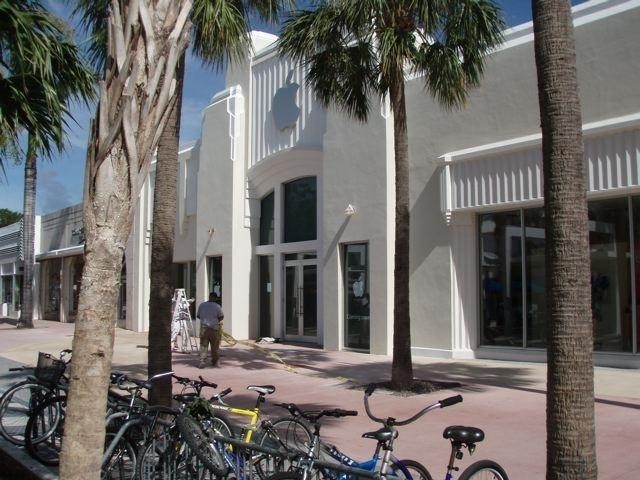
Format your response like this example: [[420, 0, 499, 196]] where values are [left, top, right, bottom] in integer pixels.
[[171, 289, 199, 353]]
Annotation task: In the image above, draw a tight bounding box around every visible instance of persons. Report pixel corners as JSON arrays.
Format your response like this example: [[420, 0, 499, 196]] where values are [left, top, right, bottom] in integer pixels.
[[169, 289, 196, 356], [196, 291, 225, 370]]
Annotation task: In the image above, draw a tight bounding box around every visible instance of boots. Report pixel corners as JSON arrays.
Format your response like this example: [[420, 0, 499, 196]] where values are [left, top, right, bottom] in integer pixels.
[[211, 358, 220, 368], [199, 359, 206, 368]]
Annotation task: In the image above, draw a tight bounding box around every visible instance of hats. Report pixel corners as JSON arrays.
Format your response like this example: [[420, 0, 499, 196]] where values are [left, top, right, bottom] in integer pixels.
[[209, 292, 222, 298]]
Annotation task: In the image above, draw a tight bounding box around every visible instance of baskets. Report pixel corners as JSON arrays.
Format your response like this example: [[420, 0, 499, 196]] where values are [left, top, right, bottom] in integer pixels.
[[34, 352, 64, 385]]
